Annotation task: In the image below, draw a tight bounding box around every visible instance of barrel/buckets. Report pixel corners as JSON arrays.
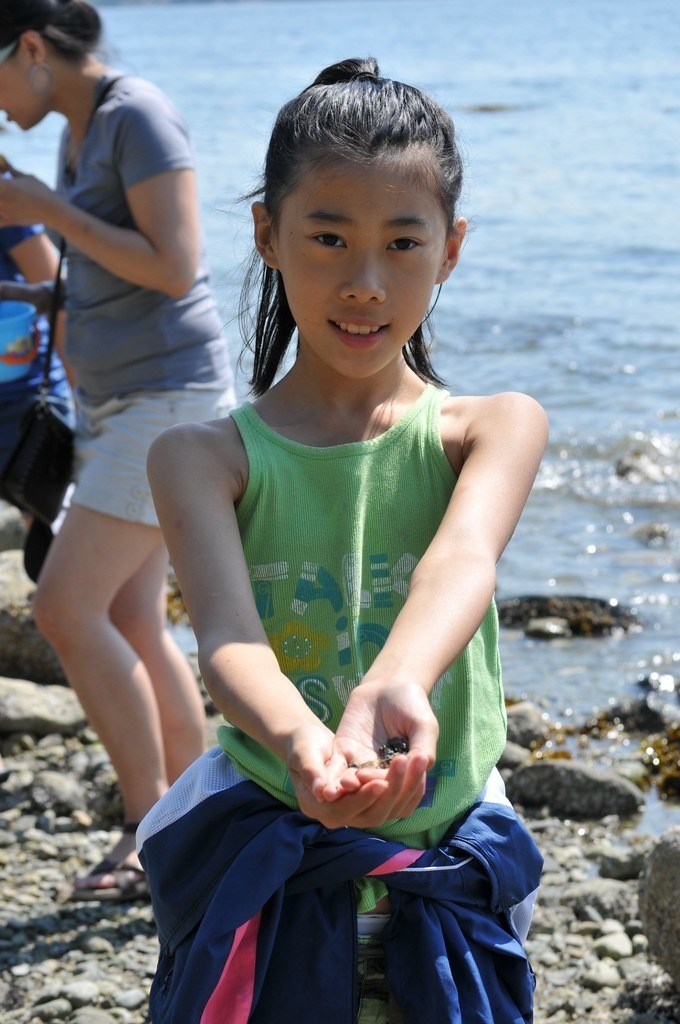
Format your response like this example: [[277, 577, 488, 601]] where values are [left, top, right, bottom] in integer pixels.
[[0, 300, 41, 382]]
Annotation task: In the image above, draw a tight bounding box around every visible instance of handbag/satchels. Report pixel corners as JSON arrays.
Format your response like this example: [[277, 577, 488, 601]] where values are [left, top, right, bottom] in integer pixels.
[[0, 404, 76, 525]]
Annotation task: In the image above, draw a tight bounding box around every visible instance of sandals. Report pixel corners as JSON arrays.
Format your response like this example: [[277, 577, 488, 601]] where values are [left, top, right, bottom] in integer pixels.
[[70, 823, 153, 899]]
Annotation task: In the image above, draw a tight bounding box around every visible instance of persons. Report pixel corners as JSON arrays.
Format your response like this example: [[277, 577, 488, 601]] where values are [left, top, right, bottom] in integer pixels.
[[0, 0, 241, 901], [0, 171, 76, 586], [135, 57, 550, 1024]]
